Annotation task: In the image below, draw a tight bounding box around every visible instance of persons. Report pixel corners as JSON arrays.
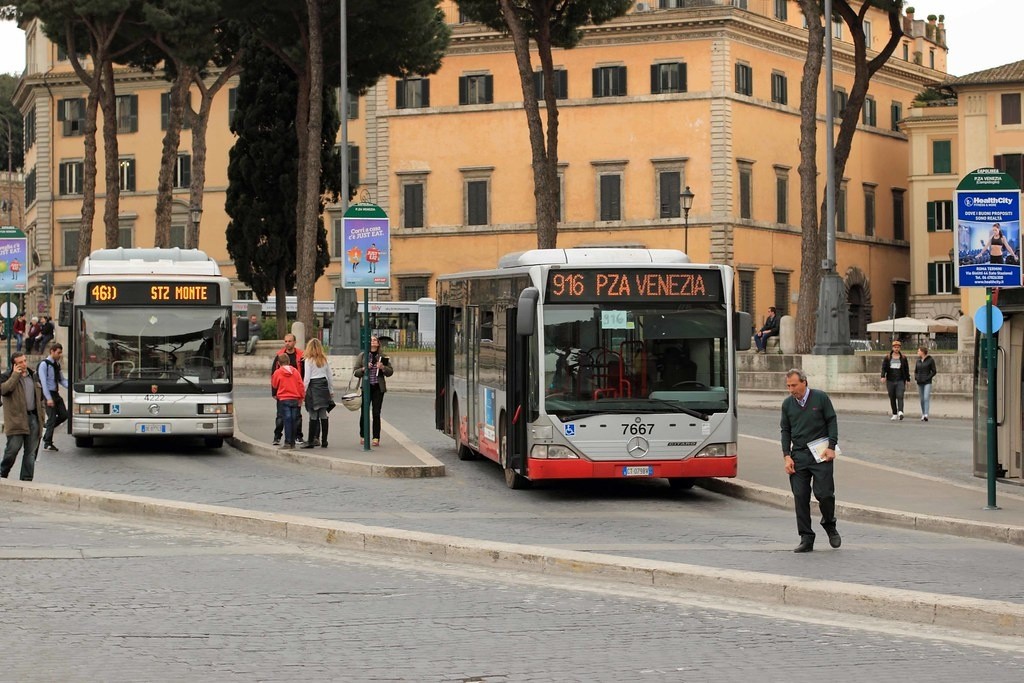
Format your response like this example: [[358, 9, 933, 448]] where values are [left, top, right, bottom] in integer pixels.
[[914, 347, 937, 422], [272, 353, 305, 448], [378, 319, 396, 329], [0, 320, 6, 340], [25, 316, 54, 355], [14, 312, 26, 352], [610, 343, 697, 399], [0, 352, 45, 482], [352, 338, 393, 445], [244, 315, 260, 355], [753, 308, 780, 354], [270, 334, 305, 445], [881, 341, 910, 420], [780, 370, 841, 552], [37, 344, 67, 452], [975, 224, 1018, 265], [300, 339, 335, 449]]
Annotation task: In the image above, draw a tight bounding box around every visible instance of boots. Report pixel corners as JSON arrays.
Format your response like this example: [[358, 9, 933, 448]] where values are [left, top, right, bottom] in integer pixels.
[[300, 419, 317, 449], [321, 419, 328, 448]]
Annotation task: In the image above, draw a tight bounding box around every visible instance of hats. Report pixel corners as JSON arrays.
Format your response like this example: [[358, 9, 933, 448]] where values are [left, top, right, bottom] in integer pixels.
[[892, 341, 901, 346]]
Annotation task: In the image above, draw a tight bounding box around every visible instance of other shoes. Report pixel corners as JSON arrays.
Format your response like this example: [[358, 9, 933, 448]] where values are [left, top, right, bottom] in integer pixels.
[[751, 348, 758, 354], [244, 352, 250, 356], [921, 414, 928, 421], [372, 438, 380, 445], [296, 438, 304, 444], [278, 444, 291, 449], [759, 349, 766, 354], [24, 351, 31, 355], [273, 440, 279, 444], [360, 439, 364, 446], [314, 441, 320, 446], [35, 351, 43, 355], [44, 443, 59, 452]]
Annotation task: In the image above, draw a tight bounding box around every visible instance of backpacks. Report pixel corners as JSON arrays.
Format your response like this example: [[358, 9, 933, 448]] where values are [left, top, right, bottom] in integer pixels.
[[33, 358, 58, 401]]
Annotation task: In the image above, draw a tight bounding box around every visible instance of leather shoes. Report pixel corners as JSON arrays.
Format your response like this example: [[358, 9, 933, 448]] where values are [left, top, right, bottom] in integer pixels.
[[828, 529, 841, 547], [794, 542, 813, 553]]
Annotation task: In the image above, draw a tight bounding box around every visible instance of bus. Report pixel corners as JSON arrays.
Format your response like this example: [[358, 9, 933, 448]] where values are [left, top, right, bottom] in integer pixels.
[[435, 243, 752, 492], [233, 298, 458, 348], [57, 246, 251, 449]]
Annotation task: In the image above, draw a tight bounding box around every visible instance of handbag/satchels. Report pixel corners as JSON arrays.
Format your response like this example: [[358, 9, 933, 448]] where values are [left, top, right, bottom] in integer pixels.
[[341, 370, 363, 411]]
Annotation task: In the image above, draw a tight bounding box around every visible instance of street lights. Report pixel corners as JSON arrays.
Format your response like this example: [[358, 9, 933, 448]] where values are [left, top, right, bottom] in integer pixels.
[[680, 185, 696, 254]]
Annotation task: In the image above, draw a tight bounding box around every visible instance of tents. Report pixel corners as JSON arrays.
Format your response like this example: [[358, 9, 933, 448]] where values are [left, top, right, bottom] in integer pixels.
[[865, 317, 957, 343]]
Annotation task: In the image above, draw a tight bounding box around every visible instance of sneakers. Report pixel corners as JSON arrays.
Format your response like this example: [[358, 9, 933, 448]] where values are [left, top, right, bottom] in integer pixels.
[[891, 415, 898, 420], [899, 411, 904, 420]]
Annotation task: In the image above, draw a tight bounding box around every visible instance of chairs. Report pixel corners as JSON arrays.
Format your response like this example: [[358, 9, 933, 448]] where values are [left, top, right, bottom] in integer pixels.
[[566, 347, 697, 398]]
